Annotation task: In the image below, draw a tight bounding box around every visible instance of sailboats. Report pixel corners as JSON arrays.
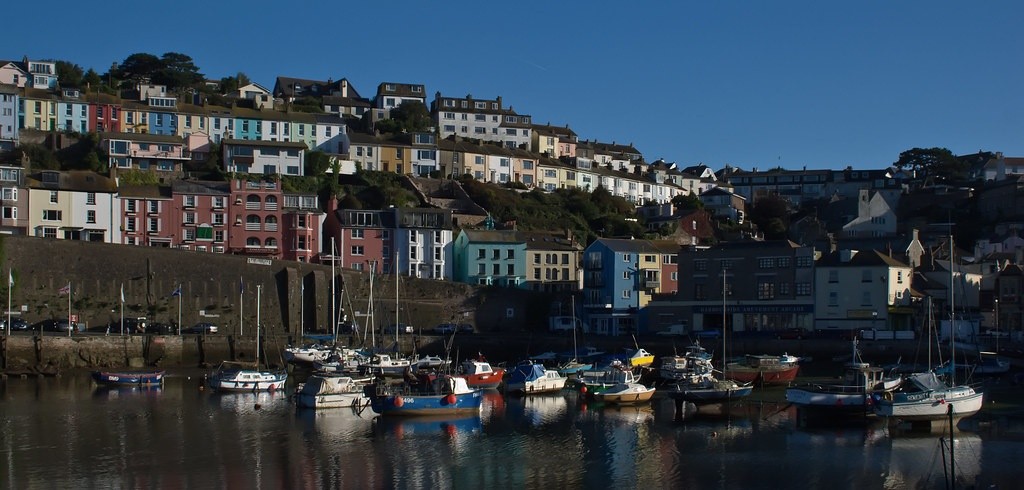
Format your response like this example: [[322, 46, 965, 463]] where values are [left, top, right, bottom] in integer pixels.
[[203, 284, 289, 394], [861, 235, 985, 427]]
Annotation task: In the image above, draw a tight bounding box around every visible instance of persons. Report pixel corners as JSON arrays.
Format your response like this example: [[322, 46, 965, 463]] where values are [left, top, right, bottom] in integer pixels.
[[73, 321, 78, 334], [126, 319, 130, 335], [174, 322, 177, 335], [105, 322, 110, 336]]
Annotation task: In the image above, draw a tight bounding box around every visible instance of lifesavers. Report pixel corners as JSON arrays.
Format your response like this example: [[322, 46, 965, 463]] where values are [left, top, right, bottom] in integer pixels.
[[404, 386, 409, 394], [441, 381, 444, 388], [837, 400, 843, 406], [884, 392, 893, 401]]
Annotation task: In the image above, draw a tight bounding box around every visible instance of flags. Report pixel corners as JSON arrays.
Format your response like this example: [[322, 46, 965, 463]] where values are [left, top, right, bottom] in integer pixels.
[[9, 272, 14, 286], [56, 284, 70, 295], [169, 288, 180, 295], [240, 278, 243, 293], [121, 287, 125, 303]]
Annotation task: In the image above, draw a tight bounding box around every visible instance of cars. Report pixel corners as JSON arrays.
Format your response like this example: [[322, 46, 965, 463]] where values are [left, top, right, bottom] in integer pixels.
[[385, 322, 410, 334], [342, 321, 360, 333], [986, 328, 1010, 337], [694, 326, 724, 339], [44, 319, 76, 333], [189, 322, 218, 334], [459, 324, 474, 333], [6, 316, 29, 332], [774, 328, 810, 339], [104, 317, 146, 335], [145, 322, 175, 334], [432, 323, 461, 334]]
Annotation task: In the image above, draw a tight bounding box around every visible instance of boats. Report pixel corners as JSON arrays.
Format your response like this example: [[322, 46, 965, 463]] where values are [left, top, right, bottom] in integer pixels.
[[784, 335, 904, 418], [282, 236, 800, 417], [503, 359, 570, 399], [91, 369, 166, 391], [592, 382, 657, 405], [724, 351, 800, 387]]
